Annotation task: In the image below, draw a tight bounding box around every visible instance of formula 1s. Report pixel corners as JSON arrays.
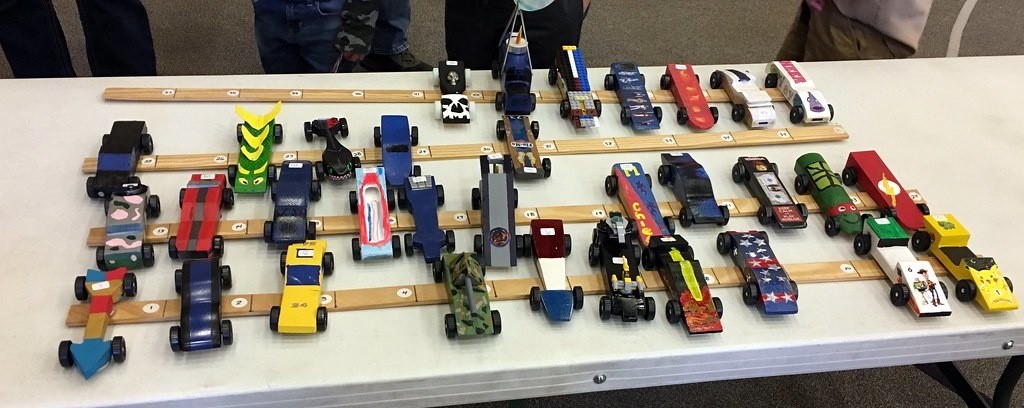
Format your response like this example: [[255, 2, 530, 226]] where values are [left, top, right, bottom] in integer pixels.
[[588, 212, 658, 323]]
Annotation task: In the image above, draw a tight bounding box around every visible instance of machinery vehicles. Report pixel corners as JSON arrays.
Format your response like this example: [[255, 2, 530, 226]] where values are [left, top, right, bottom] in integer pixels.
[[491, 0, 536, 114]]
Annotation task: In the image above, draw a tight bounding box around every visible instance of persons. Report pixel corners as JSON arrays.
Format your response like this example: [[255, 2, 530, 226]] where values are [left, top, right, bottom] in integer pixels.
[[443, 0, 593, 69], [251, 0, 436, 74], [775, 0, 933, 63], [0, 0, 155, 77]]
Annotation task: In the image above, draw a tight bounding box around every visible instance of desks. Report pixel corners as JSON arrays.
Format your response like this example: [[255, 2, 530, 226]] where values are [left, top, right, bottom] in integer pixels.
[[0, 55, 1024, 408]]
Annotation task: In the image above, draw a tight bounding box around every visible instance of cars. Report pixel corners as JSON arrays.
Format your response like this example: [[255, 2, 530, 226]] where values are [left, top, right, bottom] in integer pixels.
[[658, 153, 730, 228], [732, 156, 808, 229], [661, 63, 719, 131], [605, 63, 663, 132], [764, 61, 835, 125], [87, 121, 160, 271], [710, 68, 776, 129], [548, 45, 602, 130], [349, 114, 501, 339], [170, 100, 359, 352], [794, 149, 1018, 317], [472, 114, 583, 322], [58, 267, 137, 380], [605, 162, 724, 336], [717, 230, 798, 315], [433, 61, 475, 123]]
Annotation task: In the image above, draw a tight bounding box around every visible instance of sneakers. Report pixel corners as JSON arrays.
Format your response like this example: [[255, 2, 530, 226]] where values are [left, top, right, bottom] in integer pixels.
[[367, 49, 434, 71]]
